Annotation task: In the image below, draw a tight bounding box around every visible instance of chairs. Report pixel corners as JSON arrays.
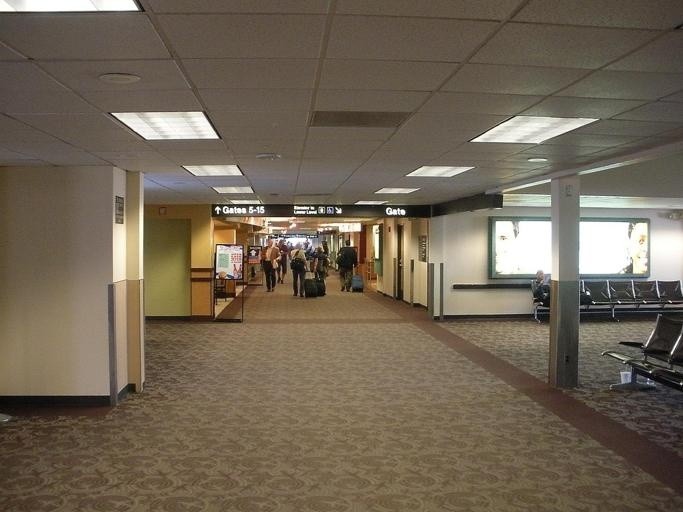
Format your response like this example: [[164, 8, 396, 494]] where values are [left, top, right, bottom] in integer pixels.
[[602, 316, 683, 395], [530, 279, 683, 323]]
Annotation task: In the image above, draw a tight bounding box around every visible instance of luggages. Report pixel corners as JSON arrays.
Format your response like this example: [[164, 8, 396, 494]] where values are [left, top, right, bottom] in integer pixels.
[[312, 277, 326, 296], [303, 279, 318, 297], [350, 275, 364, 292]]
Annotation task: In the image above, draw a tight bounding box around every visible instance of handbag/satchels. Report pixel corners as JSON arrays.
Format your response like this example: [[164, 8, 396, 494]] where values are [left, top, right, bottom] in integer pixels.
[[310, 260, 318, 272], [290, 249, 305, 270], [336, 254, 345, 265]]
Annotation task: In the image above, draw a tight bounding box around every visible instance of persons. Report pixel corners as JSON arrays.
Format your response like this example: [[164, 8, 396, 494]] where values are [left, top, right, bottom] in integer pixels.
[[580, 280, 595, 305], [261, 239, 330, 296], [496, 220, 522, 274], [337, 240, 358, 292], [533, 270, 550, 307], [619, 222, 648, 273]]
